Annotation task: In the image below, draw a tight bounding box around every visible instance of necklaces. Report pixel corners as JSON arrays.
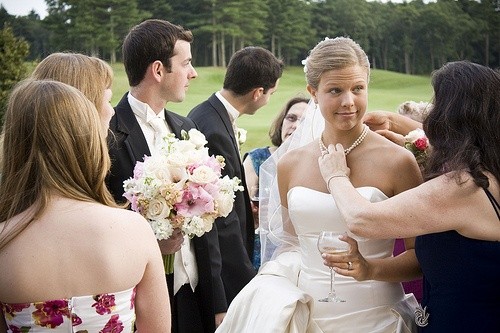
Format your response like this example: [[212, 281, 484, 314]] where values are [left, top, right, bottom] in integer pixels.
[[320, 124, 367, 152], [318, 126, 369, 155]]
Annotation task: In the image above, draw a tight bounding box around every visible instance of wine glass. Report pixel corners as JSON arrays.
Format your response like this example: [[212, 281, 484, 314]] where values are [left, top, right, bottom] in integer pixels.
[[250, 186, 270, 234], [317, 229, 352, 303]]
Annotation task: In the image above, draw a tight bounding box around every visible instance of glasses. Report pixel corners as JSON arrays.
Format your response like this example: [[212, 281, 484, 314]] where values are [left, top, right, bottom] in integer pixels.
[[284, 113, 300, 122]]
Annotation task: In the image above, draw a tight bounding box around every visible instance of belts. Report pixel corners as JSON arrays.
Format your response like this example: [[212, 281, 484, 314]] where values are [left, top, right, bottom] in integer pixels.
[[169, 282, 204, 296]]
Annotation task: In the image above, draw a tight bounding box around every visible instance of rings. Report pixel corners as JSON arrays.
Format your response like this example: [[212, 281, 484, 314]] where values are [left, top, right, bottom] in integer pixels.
[[348, 262, 352, 269], [322, 151, 328, 156]]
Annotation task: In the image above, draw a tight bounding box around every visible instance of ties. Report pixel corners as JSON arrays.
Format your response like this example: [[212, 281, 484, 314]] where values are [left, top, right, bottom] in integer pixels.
[[150, 118, 168, 137]]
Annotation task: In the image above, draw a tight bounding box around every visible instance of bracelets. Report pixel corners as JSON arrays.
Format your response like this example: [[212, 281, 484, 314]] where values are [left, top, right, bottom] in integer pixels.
[[327, 175, 349, 193]]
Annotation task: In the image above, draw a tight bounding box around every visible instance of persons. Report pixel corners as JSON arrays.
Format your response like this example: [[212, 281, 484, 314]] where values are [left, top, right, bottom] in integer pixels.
[[241, 97, 312, 276], [1, 20, 284, 333], [212, 36, 430, 333], [318, 61, 500, 333]]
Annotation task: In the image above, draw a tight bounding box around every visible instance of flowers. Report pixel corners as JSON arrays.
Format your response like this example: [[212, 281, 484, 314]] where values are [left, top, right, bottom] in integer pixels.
[[123, 128, 244, 239], [414, 137, 429, 152], [237, 127, 246, 145]]
